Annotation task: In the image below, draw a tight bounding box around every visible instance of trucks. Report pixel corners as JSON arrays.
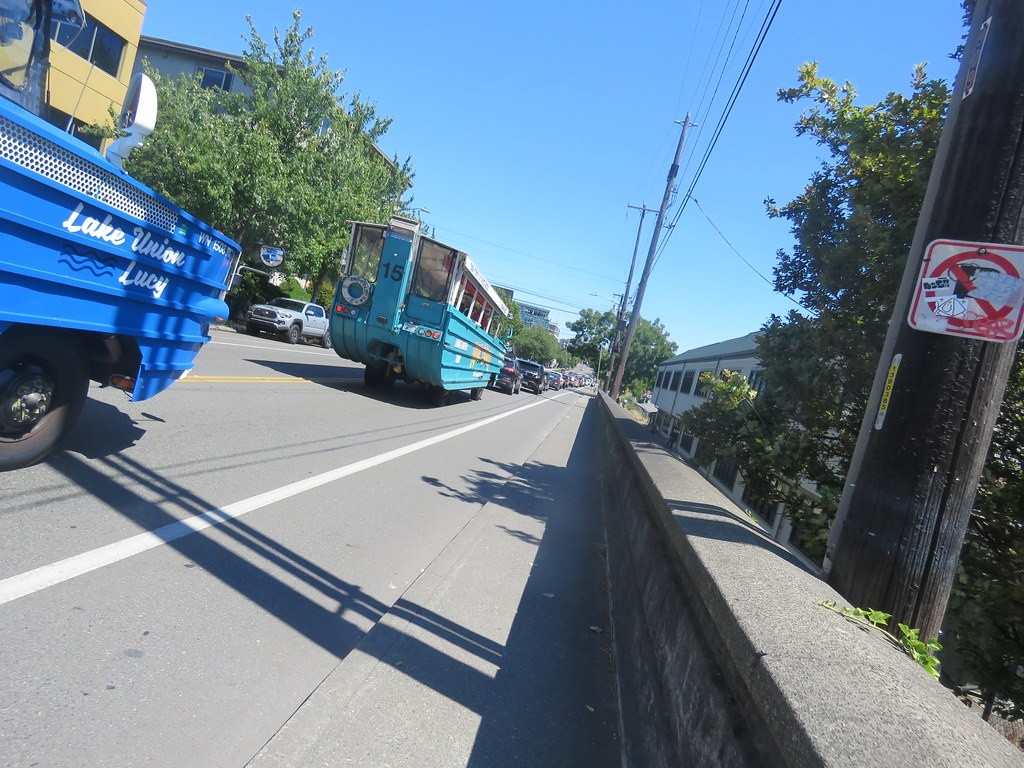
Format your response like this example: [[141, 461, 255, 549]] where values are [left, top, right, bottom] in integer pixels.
[[1, 0, 243, 470]]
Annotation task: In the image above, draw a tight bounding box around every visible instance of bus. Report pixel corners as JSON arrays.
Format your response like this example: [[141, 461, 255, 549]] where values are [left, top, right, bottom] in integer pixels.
[[329, 214, 515, 407]]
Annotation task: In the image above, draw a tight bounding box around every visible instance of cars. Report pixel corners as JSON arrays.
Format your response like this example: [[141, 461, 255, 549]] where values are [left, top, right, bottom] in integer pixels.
[[485, 356, 525, 395], [548, 369, 596, 392]]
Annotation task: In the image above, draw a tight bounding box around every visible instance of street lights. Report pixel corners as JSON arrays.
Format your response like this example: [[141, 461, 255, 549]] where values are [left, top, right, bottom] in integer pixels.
[[587, 292, 625, 354]]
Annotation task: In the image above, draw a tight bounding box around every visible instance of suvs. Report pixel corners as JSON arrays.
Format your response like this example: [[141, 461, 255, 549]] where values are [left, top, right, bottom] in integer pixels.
[[517, 357, 548, 395]]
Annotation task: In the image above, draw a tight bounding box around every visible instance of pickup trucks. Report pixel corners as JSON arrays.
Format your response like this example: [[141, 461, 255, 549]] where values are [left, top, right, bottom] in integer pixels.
[[243, 297, 331, 348]]
